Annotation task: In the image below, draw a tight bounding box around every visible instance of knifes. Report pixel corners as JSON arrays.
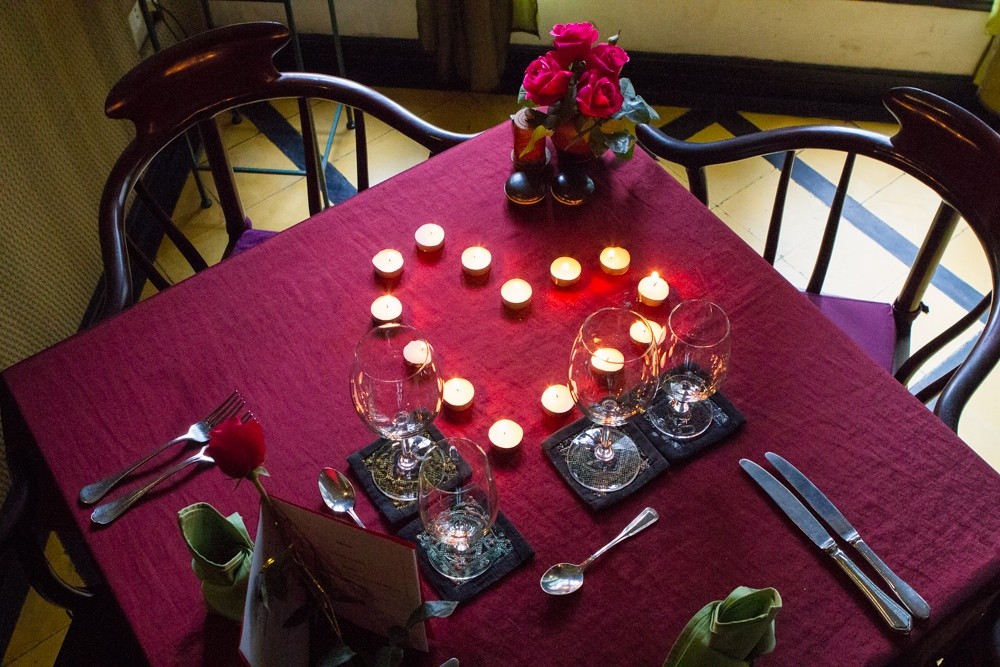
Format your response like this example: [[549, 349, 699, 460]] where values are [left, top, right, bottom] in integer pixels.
[[739, 458, 913, 632], [765, 452, 933, 619]]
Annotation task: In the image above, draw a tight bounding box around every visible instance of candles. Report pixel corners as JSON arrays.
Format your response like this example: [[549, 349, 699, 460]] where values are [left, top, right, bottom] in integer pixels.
[[372, 249, 404, 280], [590, 348, 625, 381], [443, 377, 475, 411], [637, 271, 670, 308], [401, 339, 434, 370], [370, 292, 404, 325], [414, 223, 446, 254], [599, 246, 631, 276], [488, 418, 524, 455], [550, 256, 582, 287], [540, 383, 575, 420], [460, 246, 493, 278], [501, 278, 533, 310], [629, 319, 665, 348]]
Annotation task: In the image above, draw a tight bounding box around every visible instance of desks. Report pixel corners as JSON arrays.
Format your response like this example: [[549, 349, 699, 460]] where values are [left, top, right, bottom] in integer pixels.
[[0, 117, 1000, 667]]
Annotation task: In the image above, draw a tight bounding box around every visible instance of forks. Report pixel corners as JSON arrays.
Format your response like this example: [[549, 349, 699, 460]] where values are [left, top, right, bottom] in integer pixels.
[[89, 408, 259, 524], [78, 389, 246, 505]]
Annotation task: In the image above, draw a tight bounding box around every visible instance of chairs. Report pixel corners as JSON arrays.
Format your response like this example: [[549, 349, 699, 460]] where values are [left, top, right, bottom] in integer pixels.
[[8, 21, 1000, 615]]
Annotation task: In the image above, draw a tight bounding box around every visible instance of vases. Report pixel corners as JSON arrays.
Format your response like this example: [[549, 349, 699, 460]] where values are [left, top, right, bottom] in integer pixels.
[[549, 117, 593, 156]]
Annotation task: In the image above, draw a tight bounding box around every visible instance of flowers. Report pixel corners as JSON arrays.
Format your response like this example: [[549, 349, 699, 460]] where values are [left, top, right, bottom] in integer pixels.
[[205, 415, 460, 666], [516, 22, 663, 162]]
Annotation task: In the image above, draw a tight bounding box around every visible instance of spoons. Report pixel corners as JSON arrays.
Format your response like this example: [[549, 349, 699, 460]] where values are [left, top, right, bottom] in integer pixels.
[[318, 466, 366, 531], [539, 506, 660, 596]]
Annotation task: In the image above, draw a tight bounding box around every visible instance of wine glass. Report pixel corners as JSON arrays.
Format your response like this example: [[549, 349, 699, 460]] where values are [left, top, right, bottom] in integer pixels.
[[350, 323, 445, 501], [649, 299, 734, 437], [566, 308, 660, 492], [417, 437, 499, 582]]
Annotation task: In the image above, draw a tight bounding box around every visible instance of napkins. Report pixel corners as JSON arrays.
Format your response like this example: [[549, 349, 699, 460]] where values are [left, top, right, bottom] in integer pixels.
[[176, 501, 257, 622], [662, 585, 783, 667]]
